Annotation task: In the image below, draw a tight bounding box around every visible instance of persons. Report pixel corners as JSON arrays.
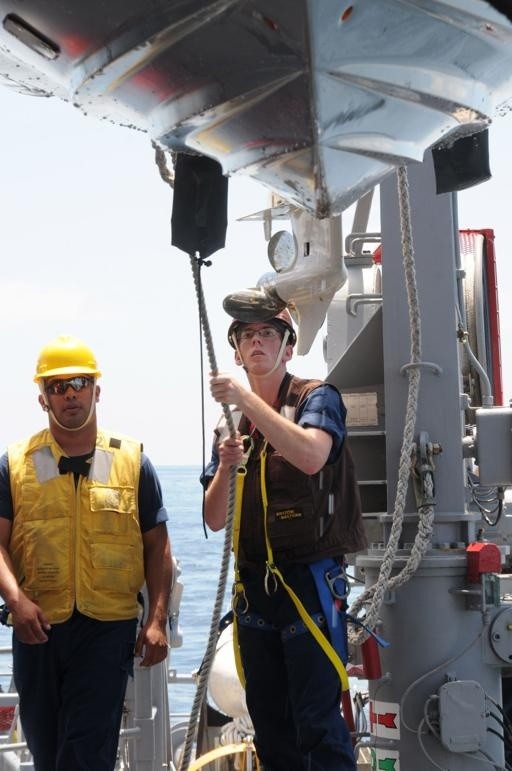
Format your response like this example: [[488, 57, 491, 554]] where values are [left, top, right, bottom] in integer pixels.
[[2, 334, 174, 771], [198, 286, 373, 770]]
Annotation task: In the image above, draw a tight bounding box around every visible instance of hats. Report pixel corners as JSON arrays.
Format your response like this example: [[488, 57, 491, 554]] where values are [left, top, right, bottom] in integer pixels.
[[31, 334, 101, 383], [227, 307, 297, 348]]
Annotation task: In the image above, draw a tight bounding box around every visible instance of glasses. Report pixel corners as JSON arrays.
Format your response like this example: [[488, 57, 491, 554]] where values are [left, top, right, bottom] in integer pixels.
[[234, 326, 281, 339], [42, 376, 93, 394]]
